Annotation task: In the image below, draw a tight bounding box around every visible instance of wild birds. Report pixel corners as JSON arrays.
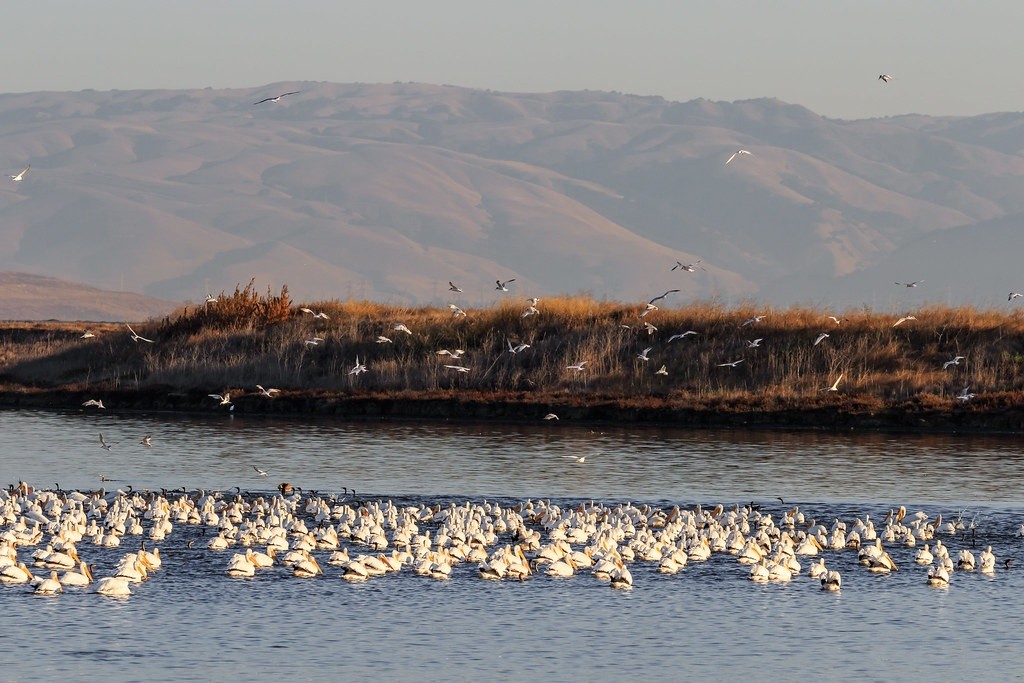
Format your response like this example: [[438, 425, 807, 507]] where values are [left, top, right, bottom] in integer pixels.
[[879, 75, 893, 83], [724, 150, 758, 164], [82, 260, 1024, 462], [3, 481, 1023, 605], [6, 165, 31, 182], [253, 92, 300, 104]]
